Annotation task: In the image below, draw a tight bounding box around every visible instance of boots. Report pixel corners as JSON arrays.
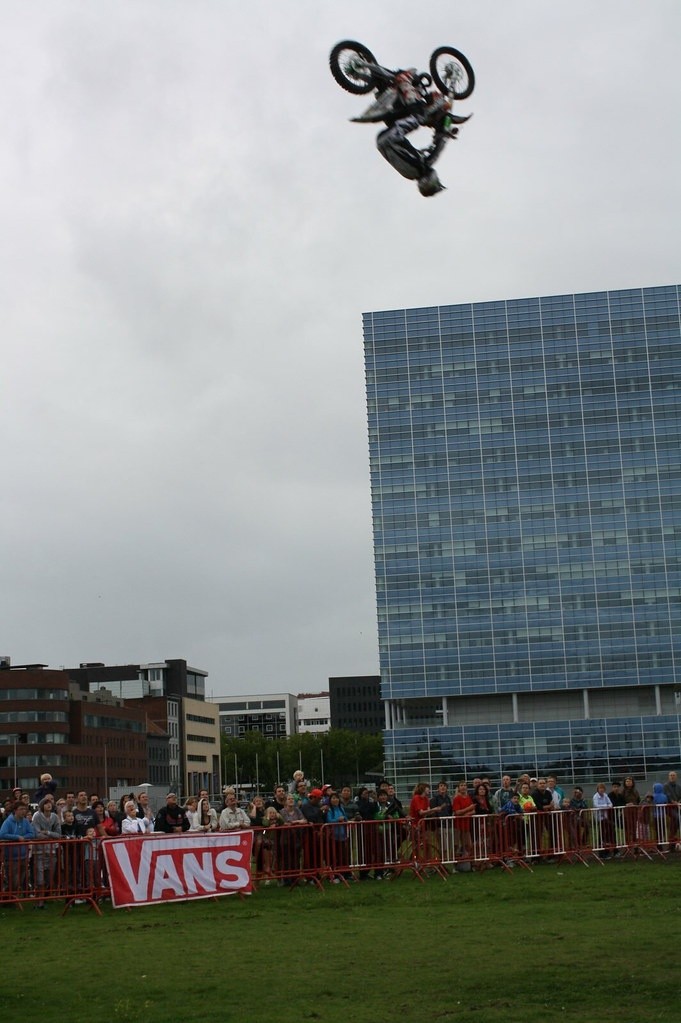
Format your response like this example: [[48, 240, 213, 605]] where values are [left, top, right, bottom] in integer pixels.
[[397, 68, 423, 107]]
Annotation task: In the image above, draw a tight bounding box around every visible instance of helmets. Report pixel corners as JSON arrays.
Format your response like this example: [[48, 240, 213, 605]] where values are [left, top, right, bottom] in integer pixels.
[[418, 167, 446, 197]]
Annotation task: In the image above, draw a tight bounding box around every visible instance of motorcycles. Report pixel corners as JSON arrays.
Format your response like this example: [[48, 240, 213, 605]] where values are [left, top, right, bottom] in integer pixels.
[[329, 40, 475, 153]]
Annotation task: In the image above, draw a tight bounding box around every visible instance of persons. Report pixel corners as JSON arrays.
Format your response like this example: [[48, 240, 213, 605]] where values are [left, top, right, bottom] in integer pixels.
[[376, 68, 450, 196], [593, 770, 681, 859], [0, 770, 407, 905], [410, 772, 588, 876]]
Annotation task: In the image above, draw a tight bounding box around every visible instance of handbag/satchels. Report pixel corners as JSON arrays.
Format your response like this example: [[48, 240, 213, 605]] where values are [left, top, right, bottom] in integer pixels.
[[397, 832, 414, 860]]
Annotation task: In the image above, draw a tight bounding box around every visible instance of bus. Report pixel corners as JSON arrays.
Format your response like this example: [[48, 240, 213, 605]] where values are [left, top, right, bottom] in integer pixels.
[[223, 783, 265, 800], [365, 772, 384, 783]]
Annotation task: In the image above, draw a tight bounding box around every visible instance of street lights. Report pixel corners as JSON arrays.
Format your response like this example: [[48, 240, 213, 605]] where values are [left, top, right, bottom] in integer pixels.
[[102, 742, 114, 802], [10, 733, 24, 788], [353, 736, 361, 786]]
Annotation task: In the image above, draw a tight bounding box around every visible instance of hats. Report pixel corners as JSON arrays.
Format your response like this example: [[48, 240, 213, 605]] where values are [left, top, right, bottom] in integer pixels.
[[530, 778, 538, 782], [323, 785, 331, 793], [13, 787, 21, 793], [39, 799, 49, 809], [309, 789, 323, 799], [223, 788, 235, 794]]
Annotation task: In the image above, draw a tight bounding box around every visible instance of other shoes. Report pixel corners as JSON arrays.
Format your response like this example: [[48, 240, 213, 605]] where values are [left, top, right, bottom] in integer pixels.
[[376, 876, 382, 880], [310, 881, 314, 886], [368, 874, 375, 879], [299, 878, 307, 885], [333, 878, 340, 884]]
